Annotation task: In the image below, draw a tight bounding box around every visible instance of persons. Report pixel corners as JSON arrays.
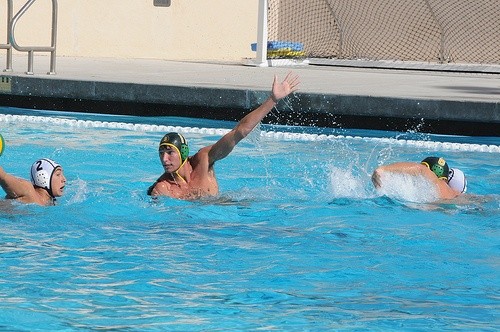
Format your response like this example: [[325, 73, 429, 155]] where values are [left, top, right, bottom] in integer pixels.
[[0, 158, 66, 207], [372, 157, 459, 200], [149, 71, 301, 198]]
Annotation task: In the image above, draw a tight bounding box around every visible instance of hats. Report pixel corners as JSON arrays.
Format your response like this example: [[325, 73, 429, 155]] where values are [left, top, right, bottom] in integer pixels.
[[421, 156, 449, 182], [159, 132, 189, 173], [446, 168, 468, 194], [30, 158, 62, 201]]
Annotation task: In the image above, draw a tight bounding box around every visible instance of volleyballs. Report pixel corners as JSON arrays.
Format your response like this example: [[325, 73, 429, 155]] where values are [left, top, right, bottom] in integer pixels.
[[0, 133, 5, 158], [447, 167, 467, 194]]
